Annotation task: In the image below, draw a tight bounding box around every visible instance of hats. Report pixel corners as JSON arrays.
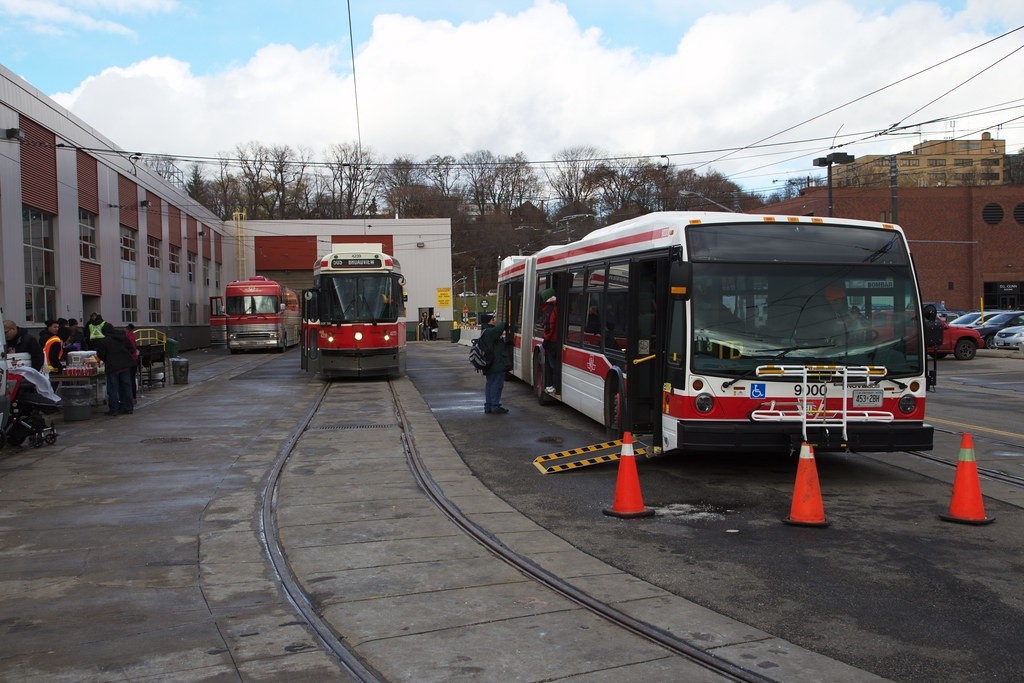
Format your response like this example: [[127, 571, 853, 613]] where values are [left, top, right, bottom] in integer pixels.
[[481, 314, 494, 324], [542, 288, 555, 301], [68, 318, 78, 327], [825, 286, 845, 300]]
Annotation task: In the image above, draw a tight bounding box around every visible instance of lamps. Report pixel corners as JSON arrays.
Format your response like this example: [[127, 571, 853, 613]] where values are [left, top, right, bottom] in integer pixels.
[[200, 231, 207, 237], [6, 127, 27, 139], [417, 243, 424, 247], [140, 200, 152, 207]]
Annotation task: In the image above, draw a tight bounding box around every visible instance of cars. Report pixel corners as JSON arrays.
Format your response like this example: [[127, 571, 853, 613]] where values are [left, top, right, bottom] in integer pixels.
[[949, 311, 1024, 350], [860, 310, 985, 360], [905, 302, 960, 324]]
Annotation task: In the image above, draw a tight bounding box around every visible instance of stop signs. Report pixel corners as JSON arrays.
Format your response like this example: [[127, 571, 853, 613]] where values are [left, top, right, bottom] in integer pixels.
[[463, 306, 468, 313]]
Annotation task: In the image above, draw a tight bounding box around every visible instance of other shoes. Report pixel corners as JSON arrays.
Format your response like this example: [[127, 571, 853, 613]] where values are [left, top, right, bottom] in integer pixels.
[[494, 407, 509, 414], [548, 388, 556, 393], [485, 409, 491, 413], [119, 409, 133, 414], [104, 411, 117, 417], [546, 386, 552, 390]]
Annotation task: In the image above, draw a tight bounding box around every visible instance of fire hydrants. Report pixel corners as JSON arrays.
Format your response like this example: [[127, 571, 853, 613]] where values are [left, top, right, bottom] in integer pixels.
[[453, 320, 459, 329]]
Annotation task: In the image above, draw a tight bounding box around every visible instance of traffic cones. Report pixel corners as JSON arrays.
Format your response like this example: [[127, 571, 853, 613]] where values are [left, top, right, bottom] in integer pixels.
[[782, 441, 830, 527], [939, 431, 996, 526], [602, 431, 655, 518]]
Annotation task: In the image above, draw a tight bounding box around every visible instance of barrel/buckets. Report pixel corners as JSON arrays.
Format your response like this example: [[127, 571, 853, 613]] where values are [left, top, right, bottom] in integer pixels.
[[0, 373, 23, 402], [172, 360, 189, 384], [59, 385, 93, 421], [136, 372, 164, 388]]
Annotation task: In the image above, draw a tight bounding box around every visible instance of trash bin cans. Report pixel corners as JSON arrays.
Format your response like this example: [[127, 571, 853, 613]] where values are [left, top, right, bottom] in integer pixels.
[[60, 385, 92, 421], [450, 329, 461, 343], [172, 360, 189, 385]]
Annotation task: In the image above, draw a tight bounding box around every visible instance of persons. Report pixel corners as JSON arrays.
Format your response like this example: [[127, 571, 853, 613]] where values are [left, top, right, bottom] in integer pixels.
[[420, 312, 439, 341], [479, 315, 511, 413], [2, 320, 43, 372], [38, 312, 139, 417], [540, 289, 559, 394]]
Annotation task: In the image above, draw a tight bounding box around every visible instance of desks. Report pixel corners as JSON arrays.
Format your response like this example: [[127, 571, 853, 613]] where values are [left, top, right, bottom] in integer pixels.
[[135, 343, 167, 390], [50, 374, 107, 408]]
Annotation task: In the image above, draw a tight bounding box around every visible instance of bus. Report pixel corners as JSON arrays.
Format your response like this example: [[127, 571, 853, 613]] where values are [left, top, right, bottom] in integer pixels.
[[496, 211, 937, 452], [301, 251, 408, 378], [208, 276, 301, 354]]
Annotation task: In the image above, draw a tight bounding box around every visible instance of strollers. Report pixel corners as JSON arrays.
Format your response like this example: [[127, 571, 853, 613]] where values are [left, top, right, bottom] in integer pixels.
[[0, 366, 64, 450]]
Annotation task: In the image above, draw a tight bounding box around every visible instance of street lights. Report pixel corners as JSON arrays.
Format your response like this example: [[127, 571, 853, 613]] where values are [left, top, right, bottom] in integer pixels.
[[348, 166, 372, 218], [658, 153, 675, 211], [342, 162, 366, 218]]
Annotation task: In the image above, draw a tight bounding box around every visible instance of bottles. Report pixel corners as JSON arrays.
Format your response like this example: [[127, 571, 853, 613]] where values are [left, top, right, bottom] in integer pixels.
[[61, 360, 99, 377], [7, 353, 31, 373]]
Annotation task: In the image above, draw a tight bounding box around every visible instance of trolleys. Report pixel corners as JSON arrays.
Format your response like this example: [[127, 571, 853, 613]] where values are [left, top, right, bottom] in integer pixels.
[[49, 370, 107, 410], [136, 344, 166, 392]]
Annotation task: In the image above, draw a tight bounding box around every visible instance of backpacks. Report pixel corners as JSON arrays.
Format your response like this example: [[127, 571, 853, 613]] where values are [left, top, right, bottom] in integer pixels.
[[469, 330, 494, 372]]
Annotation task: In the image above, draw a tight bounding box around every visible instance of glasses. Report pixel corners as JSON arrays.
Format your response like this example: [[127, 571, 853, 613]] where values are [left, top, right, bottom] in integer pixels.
[[5, 329, 10, 334]]
